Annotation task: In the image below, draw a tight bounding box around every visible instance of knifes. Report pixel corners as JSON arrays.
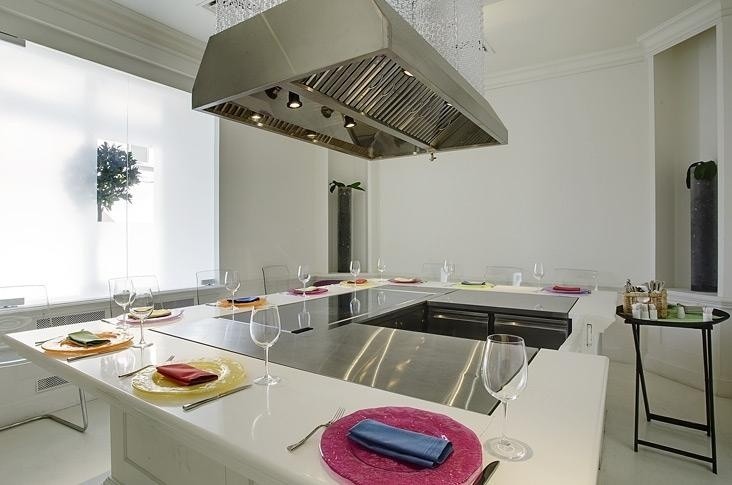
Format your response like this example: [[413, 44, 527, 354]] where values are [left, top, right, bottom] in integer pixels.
[[471, 461, 499, 484], [66, 347, 128, 361], [182, 384, 251, 412]]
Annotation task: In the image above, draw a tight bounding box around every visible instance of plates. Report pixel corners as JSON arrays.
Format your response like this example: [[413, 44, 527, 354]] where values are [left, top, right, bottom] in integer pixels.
[[542, 284, 590, 294], [42, 331, 134, 353], [320, 406, 483, 485], [132, 358, 246, 402], [217, 298, 261, 306], [388, 278, 422, 284], [454, 282, 495, 290], [340, 279, 373, 287], [117, 309, 183, 322], [289, 287, 328, 295]]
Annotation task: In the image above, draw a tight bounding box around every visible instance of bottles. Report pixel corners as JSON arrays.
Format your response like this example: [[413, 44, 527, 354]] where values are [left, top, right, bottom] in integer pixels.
[[677, 305, 685, 319], [631, 303, 658, 322]]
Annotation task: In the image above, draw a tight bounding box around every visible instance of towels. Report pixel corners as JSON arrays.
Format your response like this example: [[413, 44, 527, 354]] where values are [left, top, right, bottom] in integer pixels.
[[67, 330, 111, 347], [128, 307, 171, 319], [553, 285, 580, 292], [156, 363, 218, 386], [394, 277, 413, 283], [347, 419, 453, 469], [461, 280, 485, 285], [347, 279, 365, 284], [295, 286, 319, 292], [227, 296, 259, 303]]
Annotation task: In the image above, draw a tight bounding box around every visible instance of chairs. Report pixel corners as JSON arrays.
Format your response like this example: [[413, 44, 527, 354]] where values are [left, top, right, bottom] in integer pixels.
[[551, 269, 597, 289], [0, 283, 89, 433], [484, 267, 526, 286], [422, 263, 455, 283], [108, 275, 163, 319], [194, 269, 231, 305], [262, 265, 290, 294]]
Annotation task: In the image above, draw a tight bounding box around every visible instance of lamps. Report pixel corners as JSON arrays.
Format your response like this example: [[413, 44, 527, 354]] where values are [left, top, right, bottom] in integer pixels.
[[264, 86, 282, 100], [287, 91, 302, 109], [344, 115, 357, 128], [321, 104, 334, 119]]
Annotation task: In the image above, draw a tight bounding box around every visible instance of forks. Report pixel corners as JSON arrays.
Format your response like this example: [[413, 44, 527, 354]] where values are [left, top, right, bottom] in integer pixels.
[[287, 407, 345, 451], [119, 354, 175, 378]]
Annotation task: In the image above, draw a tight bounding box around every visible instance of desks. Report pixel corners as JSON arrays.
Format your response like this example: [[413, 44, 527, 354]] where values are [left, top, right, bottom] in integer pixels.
[[616, 305, 730, 474]]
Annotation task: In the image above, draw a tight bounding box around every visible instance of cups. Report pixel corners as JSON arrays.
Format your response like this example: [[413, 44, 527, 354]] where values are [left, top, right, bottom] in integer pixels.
[[703, 312, 712, 323]]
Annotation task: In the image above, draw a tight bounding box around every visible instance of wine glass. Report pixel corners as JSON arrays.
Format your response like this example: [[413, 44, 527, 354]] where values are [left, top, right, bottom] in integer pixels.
[[377, 258, 386, 282], [298, 264, 311, 298], [224, 271, 242, 312], [350, 260, 360, 289], [481, 334, 534, 461], [129, 287, 155, 348], [113, 279, 133, 335], [441, 257, 455, 288], [250, 304, 282, 386]]
